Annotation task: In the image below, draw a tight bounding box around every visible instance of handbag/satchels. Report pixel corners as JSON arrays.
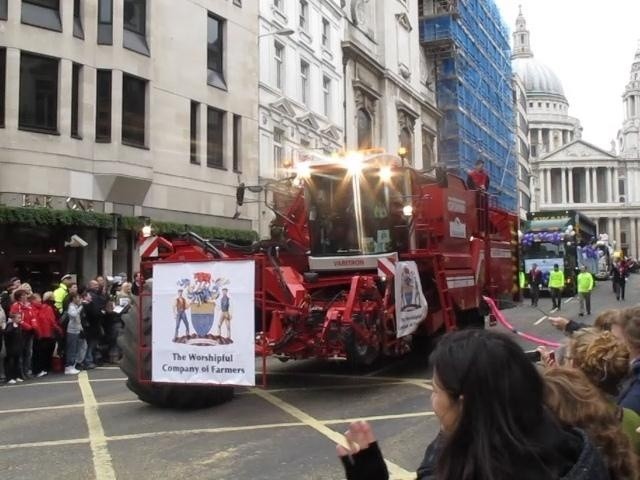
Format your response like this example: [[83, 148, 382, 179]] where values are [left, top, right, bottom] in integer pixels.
[[59, 311, 69, 328]]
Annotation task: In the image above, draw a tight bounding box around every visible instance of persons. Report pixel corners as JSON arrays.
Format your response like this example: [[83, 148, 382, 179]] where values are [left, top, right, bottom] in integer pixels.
[[517, 256, 640, 316], [1, 270, 148, 386], [534, 305, 640, 480], [335, 328, 608, 480], [467, 160, 490, 191], [217, 288, 232, 340], [173, 289, 190, 342]]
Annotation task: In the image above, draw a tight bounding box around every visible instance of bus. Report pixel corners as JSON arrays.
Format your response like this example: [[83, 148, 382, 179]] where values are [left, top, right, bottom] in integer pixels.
[[520, 206, 599, 295], [596, 239, 614, 281]]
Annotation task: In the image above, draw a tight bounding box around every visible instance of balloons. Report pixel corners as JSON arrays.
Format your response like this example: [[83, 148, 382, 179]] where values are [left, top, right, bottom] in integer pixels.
[[519, 224, 577, 247]]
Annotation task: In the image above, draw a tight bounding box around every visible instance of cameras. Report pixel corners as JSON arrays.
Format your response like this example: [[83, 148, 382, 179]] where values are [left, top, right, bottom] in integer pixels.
[[523, 350, 541, 363]]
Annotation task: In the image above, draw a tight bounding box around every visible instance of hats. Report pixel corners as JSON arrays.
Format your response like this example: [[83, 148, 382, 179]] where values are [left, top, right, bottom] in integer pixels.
[[59, 271, 72, 281]]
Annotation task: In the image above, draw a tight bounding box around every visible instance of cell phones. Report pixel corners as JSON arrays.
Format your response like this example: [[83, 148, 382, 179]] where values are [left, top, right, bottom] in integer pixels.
[[549, 350, 555, 360]]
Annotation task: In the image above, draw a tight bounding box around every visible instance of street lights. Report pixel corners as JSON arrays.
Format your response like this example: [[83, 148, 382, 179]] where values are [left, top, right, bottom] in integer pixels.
[[255, 27, 299, 222]]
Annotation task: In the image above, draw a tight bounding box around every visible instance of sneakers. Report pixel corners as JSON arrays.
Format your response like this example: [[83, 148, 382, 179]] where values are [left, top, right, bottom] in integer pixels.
[[65, 366, 82, 375], [6, 369, 48, 384]]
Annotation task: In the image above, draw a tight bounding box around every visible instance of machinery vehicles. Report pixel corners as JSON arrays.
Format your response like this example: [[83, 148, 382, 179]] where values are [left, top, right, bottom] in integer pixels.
[[121, 146, 522, 407]]
